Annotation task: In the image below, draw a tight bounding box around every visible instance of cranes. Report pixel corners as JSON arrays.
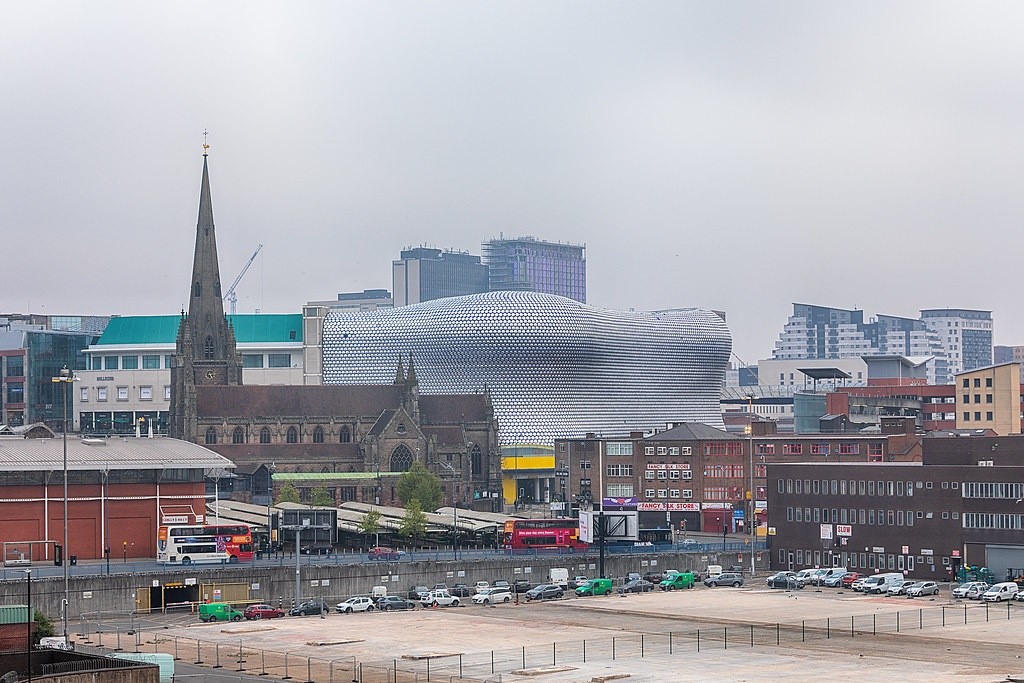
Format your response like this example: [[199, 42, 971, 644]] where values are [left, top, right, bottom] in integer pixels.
[[223, 241, 264, 340]]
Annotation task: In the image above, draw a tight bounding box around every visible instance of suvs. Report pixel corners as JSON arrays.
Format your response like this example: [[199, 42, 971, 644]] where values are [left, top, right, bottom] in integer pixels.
[[244, 605, 285, 620], [409, 580, 531, 604], [368, 547, 405, 561], [419, 591, 460, 608], [679, 539, 702, 550], [336, 596, 375, 613]]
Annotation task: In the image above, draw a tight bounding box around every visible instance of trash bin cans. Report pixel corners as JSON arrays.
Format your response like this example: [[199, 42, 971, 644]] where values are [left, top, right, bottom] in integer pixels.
[[70, 555, 77, 566], [258, 550, 262, 559]]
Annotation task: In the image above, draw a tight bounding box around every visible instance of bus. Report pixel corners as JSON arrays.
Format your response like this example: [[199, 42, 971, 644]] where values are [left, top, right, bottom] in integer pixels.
[[608, 529, 672, 553], [157, 524, 254, 566], [504, 519, 589, 555]]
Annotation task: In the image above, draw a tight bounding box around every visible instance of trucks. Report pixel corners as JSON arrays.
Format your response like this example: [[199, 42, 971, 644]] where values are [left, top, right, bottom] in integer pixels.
[[369, 586, 415, 610]]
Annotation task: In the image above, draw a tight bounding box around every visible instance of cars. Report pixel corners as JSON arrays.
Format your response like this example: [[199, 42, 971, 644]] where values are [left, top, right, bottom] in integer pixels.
[[617, 569, 701, 594]]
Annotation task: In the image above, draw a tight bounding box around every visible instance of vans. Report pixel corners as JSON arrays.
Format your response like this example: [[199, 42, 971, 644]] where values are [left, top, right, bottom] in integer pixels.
[[300, 543, 333, 555], [288, 600, 329, 616], [525, 585, 564, 600], [952, 568, 1024, 603], [199, 603, 243, 623], [703, 565, 744, 588], [766, 567, 939, 597], [547, 568, 612, 596]]
